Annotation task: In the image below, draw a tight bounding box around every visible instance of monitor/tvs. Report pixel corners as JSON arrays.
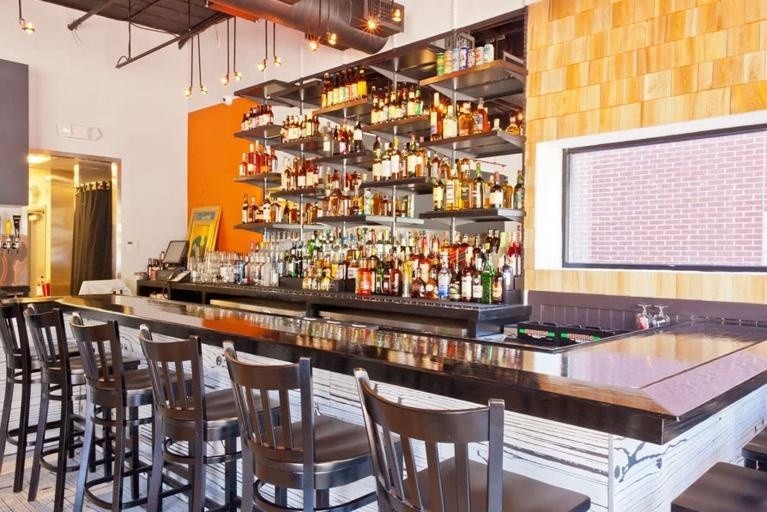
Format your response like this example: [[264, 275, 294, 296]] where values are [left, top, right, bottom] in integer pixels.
[[162, 240, 189, 266]]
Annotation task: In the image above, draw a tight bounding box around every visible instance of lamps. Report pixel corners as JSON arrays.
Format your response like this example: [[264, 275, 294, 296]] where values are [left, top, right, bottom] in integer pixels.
[[325, 0, 338, 46], [257, 19, 268, 71], [183, 35, 193, 96], [233, 13, 242, 80], [271, 21, 282, 66], [390, 1, 403, 23], [221, 16, 230, 85], [366, 1, 379, 31], [308, 0, 322, 51], [18, 0, 35, 33], [197, 31, 208, 96]]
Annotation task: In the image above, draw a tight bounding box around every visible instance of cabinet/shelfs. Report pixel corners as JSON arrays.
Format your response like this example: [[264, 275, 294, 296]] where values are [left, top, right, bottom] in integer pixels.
[[232, 59, 527, 304]]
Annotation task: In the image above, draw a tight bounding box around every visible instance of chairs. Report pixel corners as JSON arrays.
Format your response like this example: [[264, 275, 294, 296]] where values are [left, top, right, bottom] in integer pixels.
[[0, 295, 767, 511]]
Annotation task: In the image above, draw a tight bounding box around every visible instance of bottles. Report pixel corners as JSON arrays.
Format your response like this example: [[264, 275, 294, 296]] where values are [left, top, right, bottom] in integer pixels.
[[186, 66, 525, 305], [180, 302, 524, 387], [36, 275, 50, 298], [146, 250, 165, 281]]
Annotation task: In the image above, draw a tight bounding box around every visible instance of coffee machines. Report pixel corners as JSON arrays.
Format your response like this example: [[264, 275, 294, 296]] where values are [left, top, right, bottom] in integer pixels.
[[0, 205, 30, 298]]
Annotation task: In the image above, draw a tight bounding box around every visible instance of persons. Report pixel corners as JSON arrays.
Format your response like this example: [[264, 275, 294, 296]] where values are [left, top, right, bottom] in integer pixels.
[[189, 228, 210, 272]]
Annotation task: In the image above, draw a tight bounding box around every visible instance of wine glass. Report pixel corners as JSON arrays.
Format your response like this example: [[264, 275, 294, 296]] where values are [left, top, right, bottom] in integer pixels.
[[634, 303, 652, 332], [651, 305, 672, 332]]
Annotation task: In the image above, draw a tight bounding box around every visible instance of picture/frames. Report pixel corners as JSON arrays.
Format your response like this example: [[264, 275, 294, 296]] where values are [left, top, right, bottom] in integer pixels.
[[187, 220, 215, 257], [185, 204, 223, 251]]
